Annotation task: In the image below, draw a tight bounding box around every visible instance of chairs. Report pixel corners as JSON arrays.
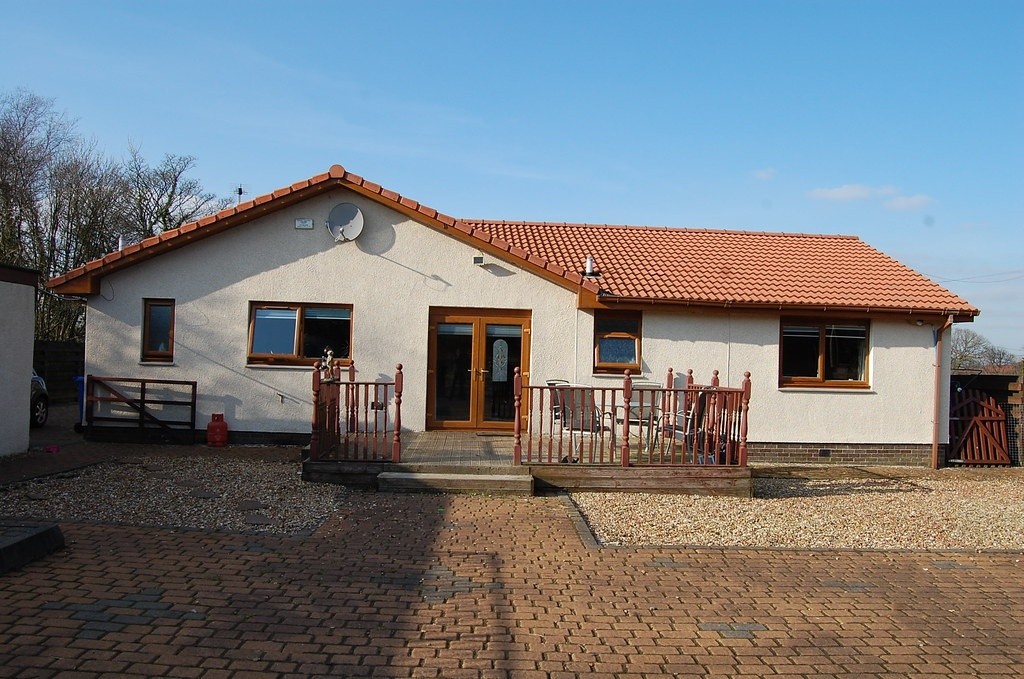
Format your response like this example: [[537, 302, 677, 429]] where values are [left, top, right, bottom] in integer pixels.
[[555, 384, 617, 462], [546, 379, 602, 456], [607, 382, 664, 459], [652, 386, 717, 463]]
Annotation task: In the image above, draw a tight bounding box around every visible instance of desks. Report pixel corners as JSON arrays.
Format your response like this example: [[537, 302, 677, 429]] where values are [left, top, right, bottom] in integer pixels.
[[574, 402, 659, 460]]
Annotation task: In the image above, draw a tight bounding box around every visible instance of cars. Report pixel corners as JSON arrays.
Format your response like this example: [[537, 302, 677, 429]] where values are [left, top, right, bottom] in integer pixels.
[[30, 366, 49, 428]]
